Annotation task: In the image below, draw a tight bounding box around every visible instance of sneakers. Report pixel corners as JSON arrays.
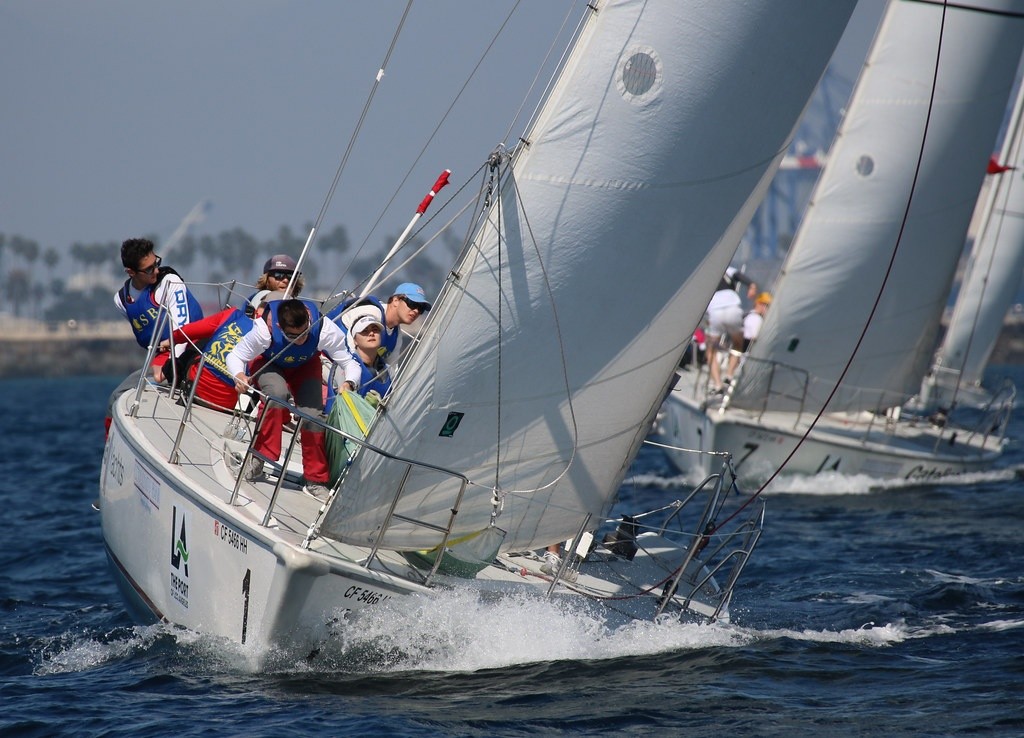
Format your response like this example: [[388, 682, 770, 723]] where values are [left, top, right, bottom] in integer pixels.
[[246, 457, 264, 478], [540, 550, 563, 576], [303, 481, 330, 503]]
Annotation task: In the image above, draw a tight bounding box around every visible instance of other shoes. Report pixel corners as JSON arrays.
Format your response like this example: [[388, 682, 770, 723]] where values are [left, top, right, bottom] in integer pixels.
[[724, 377, 732, 385], [709, 388, 724, 395]]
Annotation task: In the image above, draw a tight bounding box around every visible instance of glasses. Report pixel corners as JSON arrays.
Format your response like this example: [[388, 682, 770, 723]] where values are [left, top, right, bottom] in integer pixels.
[[135, 255, 162, 275], [401, 298, 425, 315], [267, 271, 297, 282], [281, 325, 310, 339]]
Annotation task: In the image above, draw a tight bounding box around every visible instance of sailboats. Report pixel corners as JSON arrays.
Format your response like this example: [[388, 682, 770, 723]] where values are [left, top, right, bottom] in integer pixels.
[[96, 0, 863, 678], [660, 0, 1023, 492]]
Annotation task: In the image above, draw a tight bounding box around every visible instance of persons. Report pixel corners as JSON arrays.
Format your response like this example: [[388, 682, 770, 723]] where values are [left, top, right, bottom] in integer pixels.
[[225, 299, 361, 504], [325, 313, 393, 419], [160, 295, 267, 417], [322, 282, 431, 396], [241, 254, 307, 323], [677, 269, 771, 392], [104, 238, 206, 448]]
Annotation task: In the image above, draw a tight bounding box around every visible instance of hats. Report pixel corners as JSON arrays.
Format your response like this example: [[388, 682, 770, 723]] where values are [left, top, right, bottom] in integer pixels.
[[352, 316, 384, 339], [757, 291, 771, 304], [393, 283, 431, 311], [263, 255, 302, 274]]
